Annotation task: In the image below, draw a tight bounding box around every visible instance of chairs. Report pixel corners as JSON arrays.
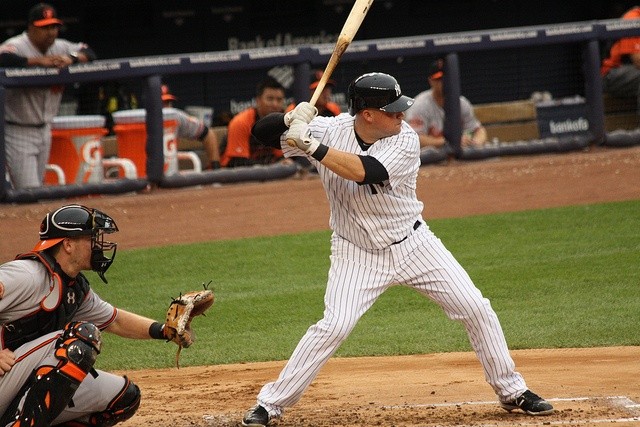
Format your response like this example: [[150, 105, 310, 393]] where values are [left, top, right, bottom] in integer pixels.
[[112, 106, 203, 179], [42, 114, 138, 185]]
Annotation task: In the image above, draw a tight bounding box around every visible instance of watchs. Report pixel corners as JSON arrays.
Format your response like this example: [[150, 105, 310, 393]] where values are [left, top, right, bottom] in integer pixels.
[[67, 50, 80, 65]]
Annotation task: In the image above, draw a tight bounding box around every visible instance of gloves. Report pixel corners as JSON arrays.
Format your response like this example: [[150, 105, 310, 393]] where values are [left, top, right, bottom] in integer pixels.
[[286, 119, 320, 157], [284, 102, 318, 128]]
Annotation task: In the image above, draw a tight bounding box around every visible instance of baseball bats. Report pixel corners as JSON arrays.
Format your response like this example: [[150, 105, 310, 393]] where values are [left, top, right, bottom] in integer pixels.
[[285, 0, 373, 147]]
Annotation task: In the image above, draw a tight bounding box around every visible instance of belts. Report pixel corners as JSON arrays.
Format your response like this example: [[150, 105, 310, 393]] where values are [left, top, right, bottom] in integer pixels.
[[393, 220, 421, 244]]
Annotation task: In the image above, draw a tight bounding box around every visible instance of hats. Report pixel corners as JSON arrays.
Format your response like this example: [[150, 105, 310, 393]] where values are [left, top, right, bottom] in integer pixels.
[[30, 4, 63, 27], [310, 68, 335, 90], [161, 84, 176, 101], [429, 60, 443, 80], [347, 72, 414, 116]]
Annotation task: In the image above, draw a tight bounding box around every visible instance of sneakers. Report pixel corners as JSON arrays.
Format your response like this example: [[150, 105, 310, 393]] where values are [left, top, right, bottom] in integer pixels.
[[500, 390, 553, 415], [242, 404, 268, 427]]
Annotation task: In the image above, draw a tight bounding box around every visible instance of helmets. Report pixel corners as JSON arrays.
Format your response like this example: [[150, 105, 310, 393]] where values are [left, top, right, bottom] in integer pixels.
[[31, 204, 118, 284]]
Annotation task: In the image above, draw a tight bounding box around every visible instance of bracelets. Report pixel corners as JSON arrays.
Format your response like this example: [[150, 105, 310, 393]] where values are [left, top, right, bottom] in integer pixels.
[[311, 142, 329, 162], [148, 321, 169, 341]]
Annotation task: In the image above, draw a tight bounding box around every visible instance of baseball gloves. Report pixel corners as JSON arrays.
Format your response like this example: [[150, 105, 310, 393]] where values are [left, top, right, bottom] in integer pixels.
[[162, 280, 215, 371]]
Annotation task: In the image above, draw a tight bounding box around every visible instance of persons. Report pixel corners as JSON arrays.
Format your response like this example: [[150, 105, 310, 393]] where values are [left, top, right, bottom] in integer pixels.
[[1, 3, 97, 190], [403, 57, 487, 148], [242, 73, 555, 427], [160, 84, 221, 168], [600, 4, 640, 105], [0, 204, 194, 427], [219, 76, 286, 167], [285, 70, 341, 118]]
